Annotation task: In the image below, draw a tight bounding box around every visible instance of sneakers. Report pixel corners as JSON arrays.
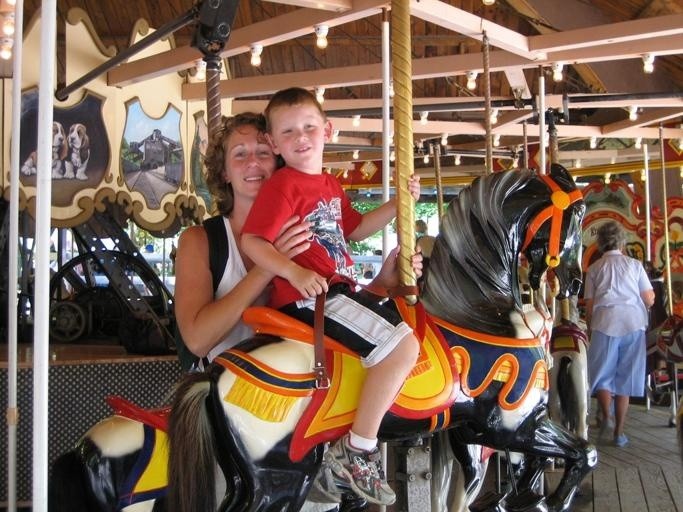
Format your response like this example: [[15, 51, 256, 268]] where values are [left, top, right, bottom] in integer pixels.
[[602, 420, 615, 430], [612, 432, 628, 447], [323, 432, 399, 509]]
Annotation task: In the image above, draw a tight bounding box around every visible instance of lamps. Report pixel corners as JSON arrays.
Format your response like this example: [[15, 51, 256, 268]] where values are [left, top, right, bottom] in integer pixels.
[[552, 63, 562, 82], [333, 83, 462, 169], [315, 26, 329, 48], [316, 85, 326, 104], [572, 133, 643, 168], [250, 42, 264, 67], [512, 156, 519, 168], [466, 70, 477, 89], [543, 130, 549, 147], [641, 53, 654, 73], [492, 108, 500, 146], [627, 105, 637, 120]]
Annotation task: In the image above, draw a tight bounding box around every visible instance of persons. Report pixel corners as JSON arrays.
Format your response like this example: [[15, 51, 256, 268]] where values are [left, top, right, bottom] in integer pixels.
[[581, 218, 656, 447], [238, 85, 422, 506], [171, 111, 314, 371]]
